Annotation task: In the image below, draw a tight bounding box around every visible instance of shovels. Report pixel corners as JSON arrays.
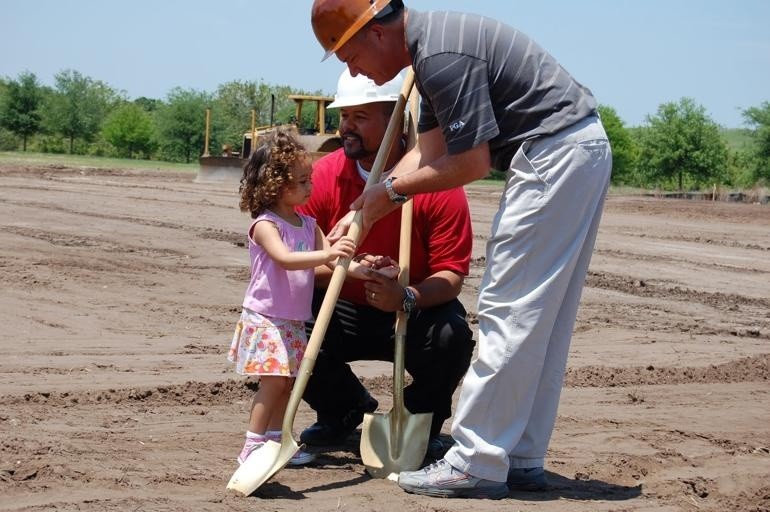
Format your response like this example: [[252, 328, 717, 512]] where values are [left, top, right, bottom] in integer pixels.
[[226, 67, 415, 497], [360, 81, 435, 479]]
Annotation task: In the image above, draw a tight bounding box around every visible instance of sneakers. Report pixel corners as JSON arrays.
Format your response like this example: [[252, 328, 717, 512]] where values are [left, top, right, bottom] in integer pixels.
[[504, 466, 548, 494], [297, 394, 380, 442], [397, 458, 509, 500], [235, 431, 318, 469]]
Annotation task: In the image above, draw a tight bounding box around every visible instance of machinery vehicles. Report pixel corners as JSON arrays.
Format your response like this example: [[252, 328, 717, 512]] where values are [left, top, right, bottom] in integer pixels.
[[195, 91, 343, 185]]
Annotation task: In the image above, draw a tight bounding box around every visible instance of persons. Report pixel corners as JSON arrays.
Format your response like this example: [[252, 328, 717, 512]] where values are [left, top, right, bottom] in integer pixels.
[[225, 132, 401, 471], [282, 62, 481, 466], [308, 0, 615, 499]]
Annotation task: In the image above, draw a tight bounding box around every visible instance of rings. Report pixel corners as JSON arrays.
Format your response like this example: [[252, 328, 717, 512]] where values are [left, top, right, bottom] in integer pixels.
[[371, 292, 376, 300]]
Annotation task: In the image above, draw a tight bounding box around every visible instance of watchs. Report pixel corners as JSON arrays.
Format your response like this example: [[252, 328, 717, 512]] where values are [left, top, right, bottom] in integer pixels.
[[400, 284, 418, 315], [385, 176, 411, 208]]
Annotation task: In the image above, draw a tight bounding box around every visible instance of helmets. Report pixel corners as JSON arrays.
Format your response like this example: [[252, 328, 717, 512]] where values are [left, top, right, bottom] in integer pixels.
[[310, 0, 392, 64], [325, 65, 407, 110]]
